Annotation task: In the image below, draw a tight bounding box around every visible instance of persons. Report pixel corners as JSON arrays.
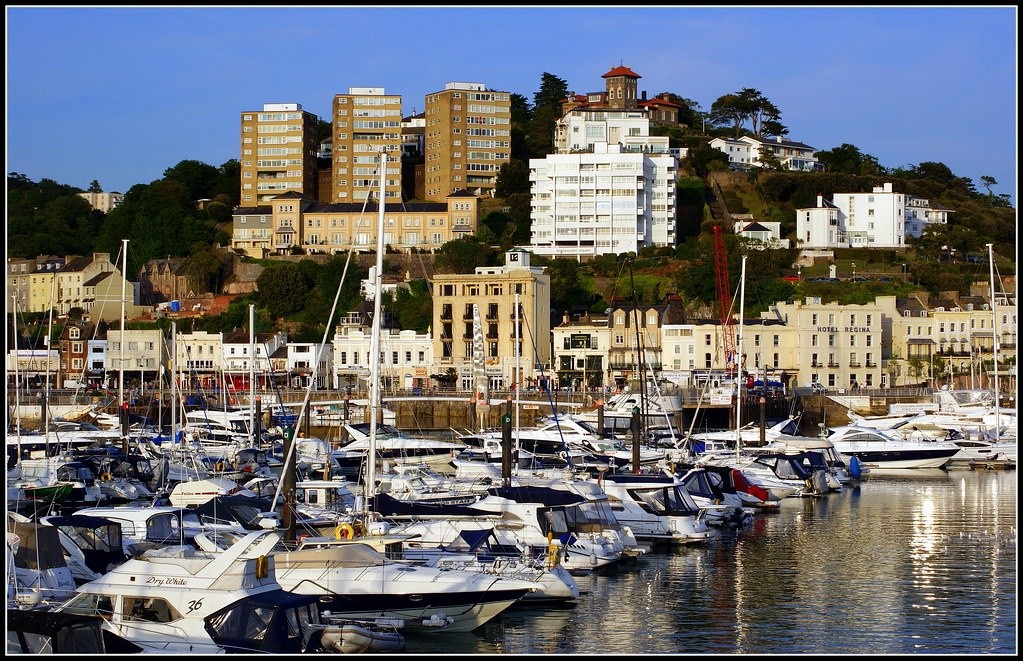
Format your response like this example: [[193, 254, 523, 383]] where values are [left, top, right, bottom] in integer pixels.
[[880, 382, 883, 389], [37, 390, 42, 404], [433, 384, 436, 390], [850, 380, 865, 393], [605, 387, 611, 392], [1002, 384, 1006, 393]]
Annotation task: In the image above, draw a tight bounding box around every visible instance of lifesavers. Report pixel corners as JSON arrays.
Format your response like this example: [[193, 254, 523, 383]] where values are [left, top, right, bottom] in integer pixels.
[[216, 462, 225, 471], [552, 549, 561, 565], [335, 524, 354, 541], [100, 472, 111, 481], [805, 480, 811, 488], [256, 555, 268, 579]]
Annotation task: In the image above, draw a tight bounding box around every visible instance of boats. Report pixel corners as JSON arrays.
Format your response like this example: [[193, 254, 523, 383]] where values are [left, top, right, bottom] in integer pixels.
[[4, 152, 1017, 656]]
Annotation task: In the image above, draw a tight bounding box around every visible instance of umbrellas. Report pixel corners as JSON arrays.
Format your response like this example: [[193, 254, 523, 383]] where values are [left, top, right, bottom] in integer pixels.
[[752, 380, 784, 387]]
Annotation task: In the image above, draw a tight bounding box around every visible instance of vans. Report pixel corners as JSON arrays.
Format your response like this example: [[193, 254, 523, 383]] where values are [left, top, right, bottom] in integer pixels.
[[803, 383, 828, 394], [63, 379, 87, 390]]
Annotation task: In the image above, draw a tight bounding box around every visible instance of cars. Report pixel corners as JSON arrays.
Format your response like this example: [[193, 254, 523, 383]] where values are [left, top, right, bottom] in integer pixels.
[[341, 385, 359, 392], [524, 385, 543, 392]]
